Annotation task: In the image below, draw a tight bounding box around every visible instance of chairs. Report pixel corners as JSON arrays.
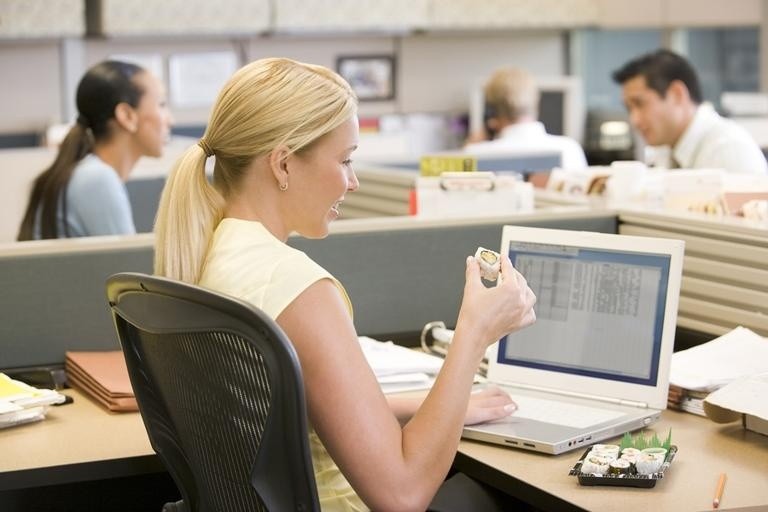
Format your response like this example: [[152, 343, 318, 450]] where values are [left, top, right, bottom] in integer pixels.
[[103, 270, 321, 510]]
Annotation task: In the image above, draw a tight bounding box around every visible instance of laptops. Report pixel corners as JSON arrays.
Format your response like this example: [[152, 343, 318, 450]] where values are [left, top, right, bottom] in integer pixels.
[[423, 223, 688, 459]]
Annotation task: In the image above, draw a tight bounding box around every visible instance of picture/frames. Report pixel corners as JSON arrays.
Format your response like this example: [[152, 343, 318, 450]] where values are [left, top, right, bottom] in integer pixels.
[[335, 54, 396, 103]]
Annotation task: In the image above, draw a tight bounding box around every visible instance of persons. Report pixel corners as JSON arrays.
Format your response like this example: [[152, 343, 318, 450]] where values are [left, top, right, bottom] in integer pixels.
[[150, 57, 539, 512], [460, 63, 589, 168], [611, 46, 768, 170], [15, 58, 176, 239]]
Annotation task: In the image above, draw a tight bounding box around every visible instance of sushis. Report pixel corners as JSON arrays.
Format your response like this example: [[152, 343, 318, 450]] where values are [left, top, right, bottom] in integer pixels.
[[581, 444, 620, 475], [609, 458, 630, 475], [621, 448, 640, 454], [475, 246, 501, 282], [621, 453, 639, 468]]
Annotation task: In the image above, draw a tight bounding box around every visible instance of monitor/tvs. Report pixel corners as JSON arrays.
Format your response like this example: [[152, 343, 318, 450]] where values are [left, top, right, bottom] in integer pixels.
[[466, 76, 587, 155]]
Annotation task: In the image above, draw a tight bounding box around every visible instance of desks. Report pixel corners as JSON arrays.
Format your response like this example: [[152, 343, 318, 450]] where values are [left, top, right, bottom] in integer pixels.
[[0, 345, 766, 511]]
